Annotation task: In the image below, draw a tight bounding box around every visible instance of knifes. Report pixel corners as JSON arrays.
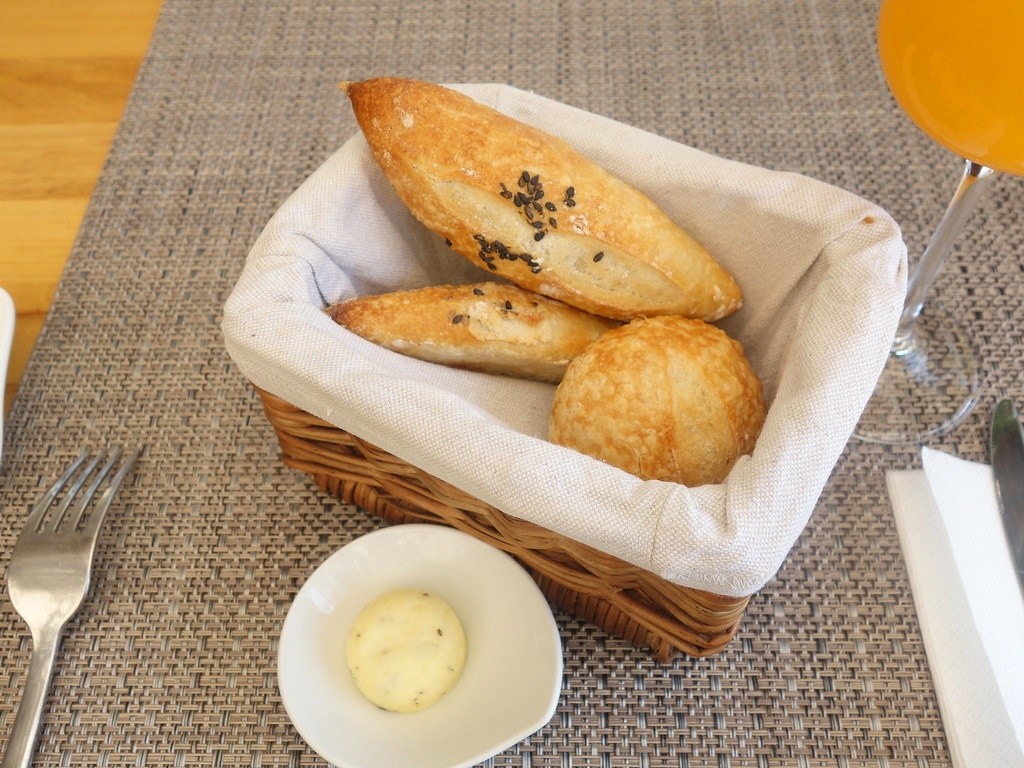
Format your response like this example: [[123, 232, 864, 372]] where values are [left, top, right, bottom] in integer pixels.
[[988, 399, 1024, 607]]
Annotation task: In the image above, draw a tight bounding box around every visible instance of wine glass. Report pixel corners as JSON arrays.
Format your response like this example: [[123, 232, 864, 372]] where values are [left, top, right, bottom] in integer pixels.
[[851, 0, 1024, 445]]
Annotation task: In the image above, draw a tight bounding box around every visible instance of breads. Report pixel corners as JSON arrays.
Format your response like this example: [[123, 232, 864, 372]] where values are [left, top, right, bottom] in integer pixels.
[[320, 280, 631, 384], [338, 78, 744, 324], [547, 314, 767, 489]]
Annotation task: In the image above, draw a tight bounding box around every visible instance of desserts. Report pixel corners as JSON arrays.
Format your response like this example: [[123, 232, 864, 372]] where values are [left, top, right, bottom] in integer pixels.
[[346, 587, 466, 713]]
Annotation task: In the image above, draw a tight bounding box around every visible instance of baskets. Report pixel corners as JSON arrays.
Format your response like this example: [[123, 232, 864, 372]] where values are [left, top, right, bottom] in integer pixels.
[[219, 80, 910, 664]]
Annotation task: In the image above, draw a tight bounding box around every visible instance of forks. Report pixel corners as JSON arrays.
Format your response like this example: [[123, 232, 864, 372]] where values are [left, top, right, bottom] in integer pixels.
[[0, 449, 143, 768]]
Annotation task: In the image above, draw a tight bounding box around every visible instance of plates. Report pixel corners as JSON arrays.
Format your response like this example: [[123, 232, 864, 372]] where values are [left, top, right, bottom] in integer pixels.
[[277, 524, 564, 768]]
